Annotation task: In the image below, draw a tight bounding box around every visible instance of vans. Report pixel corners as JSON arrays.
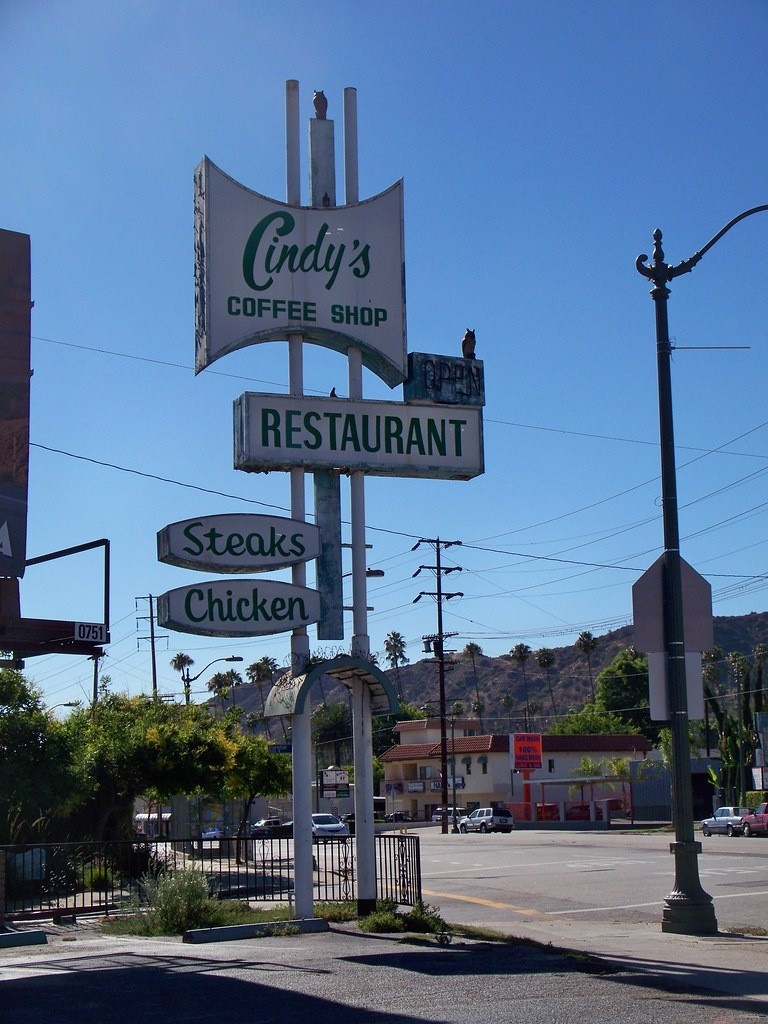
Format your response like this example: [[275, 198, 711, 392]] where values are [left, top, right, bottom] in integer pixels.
[[253, 819, 281, 829], [432, 807, 468, 823]]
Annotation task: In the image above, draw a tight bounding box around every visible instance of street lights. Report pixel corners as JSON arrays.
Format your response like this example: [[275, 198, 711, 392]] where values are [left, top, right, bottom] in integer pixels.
[[178, 654, 244, 706]]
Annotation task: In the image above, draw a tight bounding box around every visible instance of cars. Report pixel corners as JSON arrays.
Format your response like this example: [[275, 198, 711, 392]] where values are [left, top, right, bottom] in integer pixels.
[[199, 825, 269, 838], [553, 804, 603, 822], [311, 813, 349, 844], [340, 810, 415, 834], [700, 806, 756, 838], [739, 803, 768, 837]]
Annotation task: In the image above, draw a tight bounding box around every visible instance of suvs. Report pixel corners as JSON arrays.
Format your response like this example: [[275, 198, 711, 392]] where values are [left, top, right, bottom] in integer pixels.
[[458, 807, 514, 833]]
